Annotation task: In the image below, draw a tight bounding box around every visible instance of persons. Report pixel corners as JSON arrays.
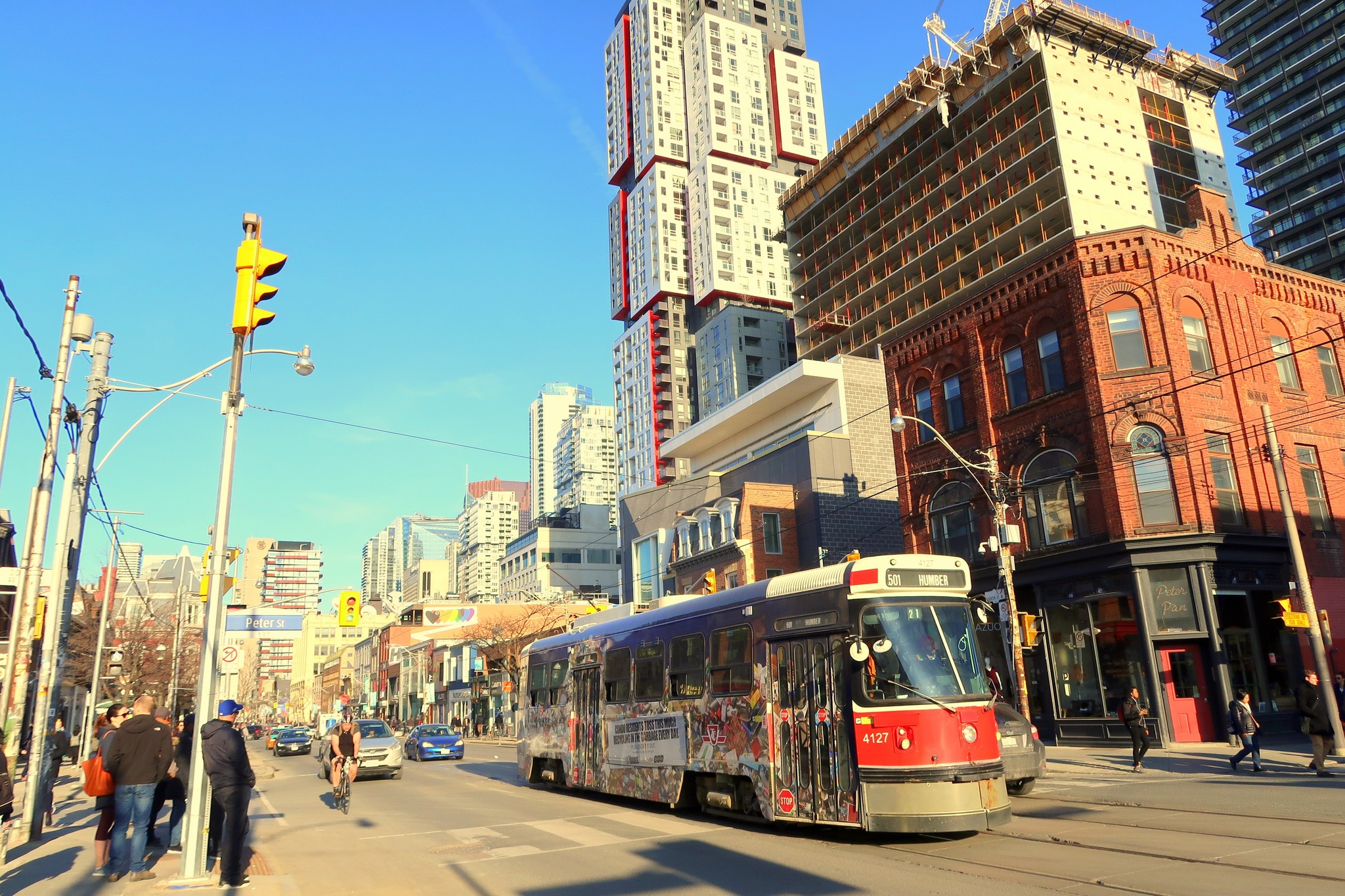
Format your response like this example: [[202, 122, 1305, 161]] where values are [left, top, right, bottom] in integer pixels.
[[22, 731, 68, 828], [1229, 689, 1268, 772], [207, 787, 225, 860], [422, 729, 445, 737], [1295, 670, 1336, 777], [200, 699, 255, 890], [366, 727, 378, 737], [329, 714, 361, 798], [387, 716, 421, 733], [495, 713, 503, 729], [984, 657, 1003, 698], [450, 712, 485, 738], [172, 721, 184, 738], [1122, 686, 1150, 773], [0, 727, 13, 815], [1334, 672, 1345, 738], [92, 696, 195, 882], [862, 618, 951, 698], [362, 716, 367, 719], [69, 725, 82, 766]]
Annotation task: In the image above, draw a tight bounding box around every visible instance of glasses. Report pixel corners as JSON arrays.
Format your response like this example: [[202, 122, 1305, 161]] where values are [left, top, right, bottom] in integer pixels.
[[231, 711, 239, 715], [112, 710, 130, 718], [1336, 677, 1343, 680]]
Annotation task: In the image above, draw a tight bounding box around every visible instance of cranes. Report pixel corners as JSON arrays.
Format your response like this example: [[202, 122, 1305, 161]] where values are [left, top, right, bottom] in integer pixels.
[[923, 0, 1010, 70]]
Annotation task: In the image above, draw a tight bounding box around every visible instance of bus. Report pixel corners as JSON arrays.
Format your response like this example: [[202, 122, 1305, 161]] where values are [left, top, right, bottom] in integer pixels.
[[517, 549, 1012, 833]]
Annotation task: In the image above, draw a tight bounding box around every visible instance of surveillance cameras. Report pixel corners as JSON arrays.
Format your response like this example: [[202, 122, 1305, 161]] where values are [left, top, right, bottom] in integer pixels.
[[978, 546, 986, 555]]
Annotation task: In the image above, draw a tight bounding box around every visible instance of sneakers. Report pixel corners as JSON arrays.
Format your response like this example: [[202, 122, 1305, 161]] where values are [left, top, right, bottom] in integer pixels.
[[235, 874, 249, 881], [219, 880, 250, 890]]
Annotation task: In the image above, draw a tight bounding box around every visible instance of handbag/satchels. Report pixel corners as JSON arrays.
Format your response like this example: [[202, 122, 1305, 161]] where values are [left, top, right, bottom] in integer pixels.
[[1302, 717, 1310, 734], [82, 730, 117, 797]]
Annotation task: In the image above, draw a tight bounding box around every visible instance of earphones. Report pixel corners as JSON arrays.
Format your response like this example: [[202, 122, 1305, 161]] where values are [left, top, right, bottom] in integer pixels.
[[1131, 693, 1133, 695]]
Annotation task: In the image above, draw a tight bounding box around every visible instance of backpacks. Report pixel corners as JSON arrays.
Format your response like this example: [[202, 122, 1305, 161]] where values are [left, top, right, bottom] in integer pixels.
[[1226, 709, 1238, 735]]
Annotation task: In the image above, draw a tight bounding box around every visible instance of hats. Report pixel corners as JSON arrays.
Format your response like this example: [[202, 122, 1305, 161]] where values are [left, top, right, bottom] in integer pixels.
[[155, 707, 174, 718], [219, 700, 243, 716], [183, 714, 194, 724]]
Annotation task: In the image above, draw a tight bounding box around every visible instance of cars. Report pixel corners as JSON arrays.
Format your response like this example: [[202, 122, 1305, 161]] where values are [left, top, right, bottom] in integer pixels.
[[992, 701, 1046, 794], [264, 723, 293, 750], [294, 723, 322, 741], [404, 724, 464, 762], [273, 729, 312, 756]]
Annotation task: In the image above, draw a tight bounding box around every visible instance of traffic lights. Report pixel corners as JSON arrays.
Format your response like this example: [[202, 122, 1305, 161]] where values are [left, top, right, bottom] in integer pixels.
[[233, 239, 288, 334], [703, 571, 717, 594], [339, 591, 360, 626], [1021, 614, 1046, 647], [200, 544, 240, 602], [1269, 598, 1292, 613]]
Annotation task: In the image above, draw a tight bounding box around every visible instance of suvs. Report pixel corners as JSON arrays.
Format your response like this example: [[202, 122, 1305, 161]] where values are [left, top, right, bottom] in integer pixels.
[[319, 717, 404, 780], [247, 725, 264, 740]]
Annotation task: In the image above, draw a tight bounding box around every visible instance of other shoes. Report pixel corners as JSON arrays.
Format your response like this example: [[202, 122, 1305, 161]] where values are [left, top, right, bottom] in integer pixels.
[[1253, 768, 1268, 772], [1229, 757, 1237, 770], [46, 814, 52, 826], [91, 868, 106, 876], [108, 871, 121, 882], [1308, 763, 1318, 770], [333, 789, 339, 797], [1133, 767, 1146, 773], [345, 788, 352, 797], [130, 870, 156, 881], [167, 845, 182, 854], [1316, 772, 1336, 777], [145, 840, 164, 849], [210, 848, 221, 860], [1132, 763, 1143, 769]]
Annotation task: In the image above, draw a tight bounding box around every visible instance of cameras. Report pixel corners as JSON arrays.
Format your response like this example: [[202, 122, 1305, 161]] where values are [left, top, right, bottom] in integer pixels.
[[1142, 709, 1146, 712]]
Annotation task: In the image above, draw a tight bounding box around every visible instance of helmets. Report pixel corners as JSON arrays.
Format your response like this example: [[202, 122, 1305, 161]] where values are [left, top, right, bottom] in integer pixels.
[[342, 714, 354, 724]]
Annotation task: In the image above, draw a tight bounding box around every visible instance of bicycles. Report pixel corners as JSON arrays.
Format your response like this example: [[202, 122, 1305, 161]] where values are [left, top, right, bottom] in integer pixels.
[[334, 756, 362, 814]]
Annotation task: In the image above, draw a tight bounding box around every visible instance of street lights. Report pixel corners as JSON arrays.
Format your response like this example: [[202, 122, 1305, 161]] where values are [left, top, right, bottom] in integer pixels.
[[341, 674, 370, 719], [891, 408, 1030, 722], [231, 578, 262, 604], [505, 589, 569, 632], [386, 644, 427, 724], [311, 685, 335, 713], [254, 689, 279, 718], [293, 687, 311, 724], [18, 329, 315, 843]]
